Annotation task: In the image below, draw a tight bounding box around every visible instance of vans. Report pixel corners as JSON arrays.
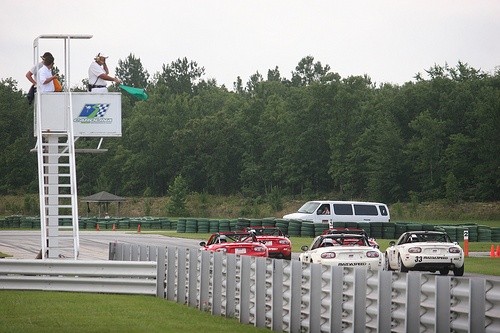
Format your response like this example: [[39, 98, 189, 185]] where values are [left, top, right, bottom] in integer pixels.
[[282, 200, 390, 224]]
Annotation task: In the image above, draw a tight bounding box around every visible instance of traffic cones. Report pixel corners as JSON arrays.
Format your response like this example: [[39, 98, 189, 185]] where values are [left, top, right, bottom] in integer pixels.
[[112, 223, 116, 231], [96, 223, 100, 231], [489, 244, 500, 257], [137, 224, 141, 232]]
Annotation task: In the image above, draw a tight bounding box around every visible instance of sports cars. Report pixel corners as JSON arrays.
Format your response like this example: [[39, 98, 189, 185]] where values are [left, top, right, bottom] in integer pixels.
[[385, 230, 465, 276], [298, 228, 389, 272], [200, 226, 292, 260]]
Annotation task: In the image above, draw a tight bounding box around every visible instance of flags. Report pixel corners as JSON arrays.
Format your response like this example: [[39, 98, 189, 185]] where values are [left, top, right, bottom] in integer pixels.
[[118, 84, 147, 101]]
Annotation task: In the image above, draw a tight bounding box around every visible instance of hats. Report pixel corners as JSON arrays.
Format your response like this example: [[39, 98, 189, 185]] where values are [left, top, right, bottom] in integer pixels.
[[97, 52, 109, 58]]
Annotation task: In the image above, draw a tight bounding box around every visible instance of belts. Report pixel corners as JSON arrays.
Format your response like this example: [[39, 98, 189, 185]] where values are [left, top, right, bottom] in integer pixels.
[[91, 84, 106, 88]]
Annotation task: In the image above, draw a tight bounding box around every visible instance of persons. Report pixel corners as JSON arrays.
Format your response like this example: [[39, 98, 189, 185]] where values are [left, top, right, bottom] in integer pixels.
[[26, 52, 52, 87], [322, 206, 329, 214], [217, 235, 227, 243], [37, 56, 58, 91], [87, 51, 122, 92]]
